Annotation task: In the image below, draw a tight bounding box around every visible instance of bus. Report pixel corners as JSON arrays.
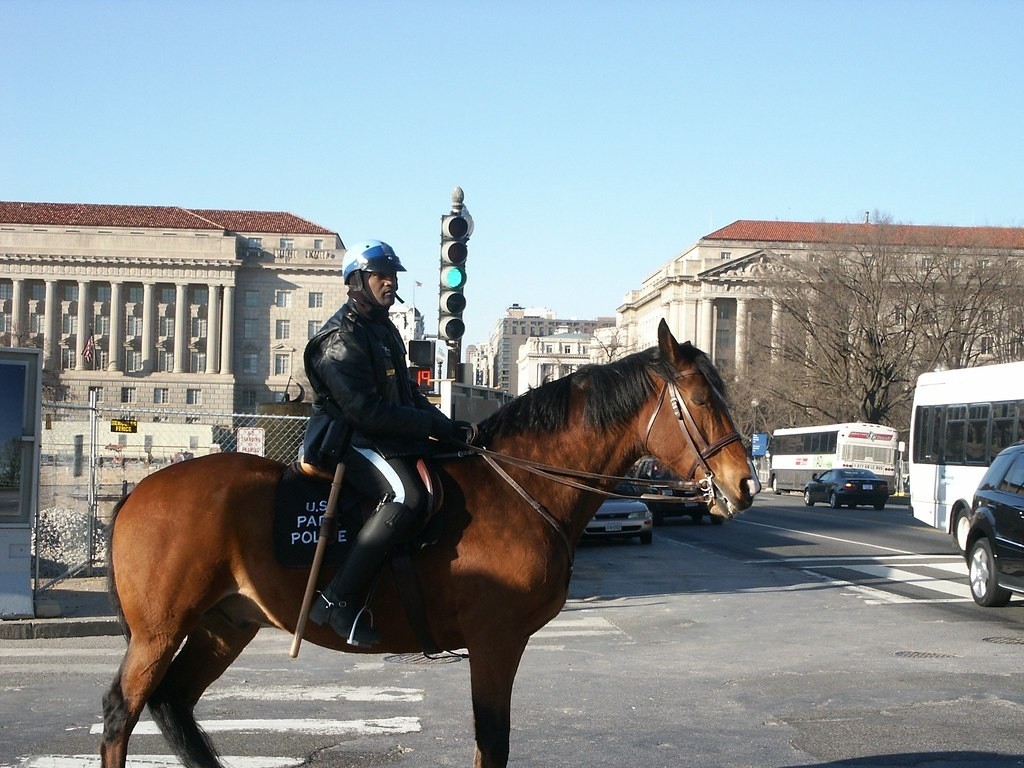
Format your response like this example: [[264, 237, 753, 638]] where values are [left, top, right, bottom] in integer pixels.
[[764, 422, 900, 496], [903, 360, 1024, 559]]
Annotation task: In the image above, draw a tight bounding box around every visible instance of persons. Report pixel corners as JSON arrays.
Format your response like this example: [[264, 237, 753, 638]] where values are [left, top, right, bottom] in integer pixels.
[[303, 240, 468, 648], [290, 444, 316, 474]]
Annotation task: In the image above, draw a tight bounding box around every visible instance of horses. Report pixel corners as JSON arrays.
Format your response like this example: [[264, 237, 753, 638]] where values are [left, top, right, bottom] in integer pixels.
[[99, 316, 764, 768]]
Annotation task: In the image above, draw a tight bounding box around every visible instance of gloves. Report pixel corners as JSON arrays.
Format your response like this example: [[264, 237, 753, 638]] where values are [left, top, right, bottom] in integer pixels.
[[431, 408, 467, 446]]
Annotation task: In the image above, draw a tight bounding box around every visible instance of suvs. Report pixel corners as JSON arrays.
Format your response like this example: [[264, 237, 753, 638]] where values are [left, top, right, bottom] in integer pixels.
[[962, 440, 1024, 608], [626, 457, 724, 526]]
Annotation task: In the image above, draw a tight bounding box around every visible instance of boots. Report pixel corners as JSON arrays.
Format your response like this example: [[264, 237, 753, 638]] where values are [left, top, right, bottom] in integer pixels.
[[308, 502, 414, 644]]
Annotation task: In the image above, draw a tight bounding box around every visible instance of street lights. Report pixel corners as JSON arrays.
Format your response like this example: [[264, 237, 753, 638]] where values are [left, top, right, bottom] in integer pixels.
[[750, 397, 760, 469], [435, 345, 445, 410]]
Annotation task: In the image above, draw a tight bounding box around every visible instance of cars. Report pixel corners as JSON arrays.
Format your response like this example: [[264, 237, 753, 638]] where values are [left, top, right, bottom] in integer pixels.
[[802, 466, 890, 511], [579, 480, 653, 545]]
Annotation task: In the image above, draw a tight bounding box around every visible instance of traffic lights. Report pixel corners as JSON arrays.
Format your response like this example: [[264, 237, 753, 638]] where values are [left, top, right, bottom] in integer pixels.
[[437, 215, 469, 342], [409, 365, 436, 392], [406, 338, 435, 367]]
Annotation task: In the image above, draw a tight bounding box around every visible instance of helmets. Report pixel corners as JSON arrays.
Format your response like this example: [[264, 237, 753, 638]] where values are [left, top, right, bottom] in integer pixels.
[[341, 239, 406, 291]]
